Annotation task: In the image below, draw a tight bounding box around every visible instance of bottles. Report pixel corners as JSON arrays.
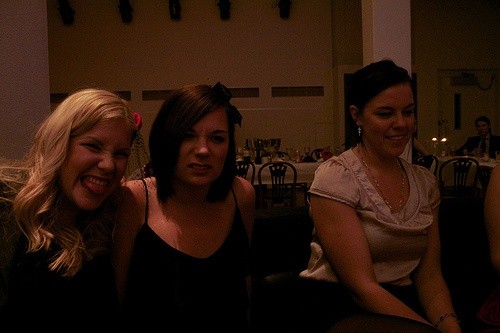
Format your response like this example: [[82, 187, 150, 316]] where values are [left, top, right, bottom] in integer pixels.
[[295, 146, 300, 163], [244, 139, 262, 164]]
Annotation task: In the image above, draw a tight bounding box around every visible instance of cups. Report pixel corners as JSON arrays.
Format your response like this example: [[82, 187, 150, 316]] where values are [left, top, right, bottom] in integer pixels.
[[484, 152, 490, 163]]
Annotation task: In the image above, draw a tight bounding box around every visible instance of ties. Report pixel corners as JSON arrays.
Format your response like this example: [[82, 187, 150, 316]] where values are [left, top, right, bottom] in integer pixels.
[[480, 137, 486, 158]]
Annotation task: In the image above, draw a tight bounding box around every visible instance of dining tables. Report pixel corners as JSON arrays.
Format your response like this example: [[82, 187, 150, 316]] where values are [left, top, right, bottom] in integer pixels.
[[236, 155, 497, 216]]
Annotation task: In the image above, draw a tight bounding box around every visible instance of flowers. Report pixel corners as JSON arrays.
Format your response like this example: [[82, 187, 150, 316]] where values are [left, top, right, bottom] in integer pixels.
[[131, 112, 143, 141]]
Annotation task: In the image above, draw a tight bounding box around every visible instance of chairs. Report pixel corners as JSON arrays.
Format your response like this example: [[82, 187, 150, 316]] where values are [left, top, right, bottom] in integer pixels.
[[426, 156, 438, 176], [439, 158, 480, 204], [258, 161, 297, 206], [233, 161, 255, 189]]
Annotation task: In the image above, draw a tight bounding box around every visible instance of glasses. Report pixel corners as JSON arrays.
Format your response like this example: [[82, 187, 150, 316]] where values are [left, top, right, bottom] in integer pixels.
[[476, 124, 486, 128]]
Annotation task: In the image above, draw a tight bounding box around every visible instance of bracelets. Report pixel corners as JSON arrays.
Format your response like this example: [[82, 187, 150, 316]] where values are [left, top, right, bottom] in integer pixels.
[[434, 313, 461, 329]]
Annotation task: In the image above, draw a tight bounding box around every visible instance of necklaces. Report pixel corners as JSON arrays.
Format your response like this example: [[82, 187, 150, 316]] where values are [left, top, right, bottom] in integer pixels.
[[358, 145, 406, 213]]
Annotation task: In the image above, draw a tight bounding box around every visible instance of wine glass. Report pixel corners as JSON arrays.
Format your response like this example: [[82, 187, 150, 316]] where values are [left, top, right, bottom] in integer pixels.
[[305, 145, 311, 164]]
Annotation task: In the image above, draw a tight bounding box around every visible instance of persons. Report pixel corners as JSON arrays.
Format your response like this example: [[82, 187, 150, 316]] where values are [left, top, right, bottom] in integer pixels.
[[0, 60, 500, 333]]
